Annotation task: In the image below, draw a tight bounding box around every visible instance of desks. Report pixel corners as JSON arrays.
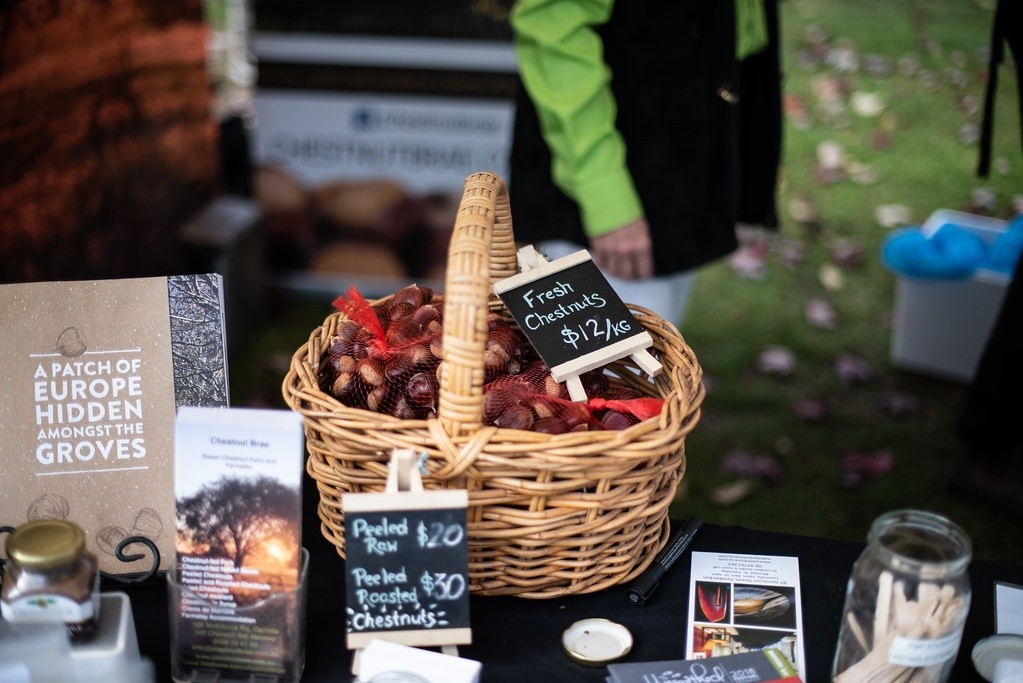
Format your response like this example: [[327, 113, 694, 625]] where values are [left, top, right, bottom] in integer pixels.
[[99, 522, 1023, 683]]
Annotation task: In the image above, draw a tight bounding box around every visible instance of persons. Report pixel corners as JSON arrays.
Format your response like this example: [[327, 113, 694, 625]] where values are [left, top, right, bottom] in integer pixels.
[[506, 1, 790, 384]]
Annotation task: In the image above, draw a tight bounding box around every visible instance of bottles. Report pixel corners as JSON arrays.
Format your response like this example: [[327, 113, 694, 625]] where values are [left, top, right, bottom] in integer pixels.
[[831, 511, 973, 683]]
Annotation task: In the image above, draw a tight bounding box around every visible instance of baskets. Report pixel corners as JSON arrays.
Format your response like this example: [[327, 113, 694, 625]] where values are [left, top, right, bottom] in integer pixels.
[[281, 171, 704, 597]]
